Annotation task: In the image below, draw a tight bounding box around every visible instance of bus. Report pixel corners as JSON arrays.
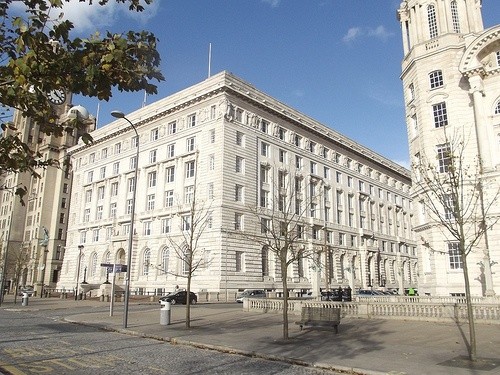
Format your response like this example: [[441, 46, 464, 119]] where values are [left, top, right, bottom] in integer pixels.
[[321, 285, 351, 302]]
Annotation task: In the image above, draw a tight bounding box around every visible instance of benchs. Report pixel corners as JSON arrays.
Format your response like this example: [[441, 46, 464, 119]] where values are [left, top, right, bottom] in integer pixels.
[[294, 306, 342, 337]]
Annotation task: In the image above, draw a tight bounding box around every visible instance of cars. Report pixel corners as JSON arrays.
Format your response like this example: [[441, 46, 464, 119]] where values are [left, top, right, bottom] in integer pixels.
[[159, 290, 198, 305], [19, 287, 34, 296], [359, 289, 398, 296]]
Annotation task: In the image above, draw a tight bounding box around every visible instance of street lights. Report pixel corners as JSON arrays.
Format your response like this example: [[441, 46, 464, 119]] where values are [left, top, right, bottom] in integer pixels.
[[75, 245, 85, 300], [111, 110, 140, 328]]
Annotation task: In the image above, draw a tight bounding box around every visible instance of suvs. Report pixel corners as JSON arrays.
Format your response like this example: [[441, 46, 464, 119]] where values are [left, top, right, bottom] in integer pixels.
[[236, 290, 267, 302]]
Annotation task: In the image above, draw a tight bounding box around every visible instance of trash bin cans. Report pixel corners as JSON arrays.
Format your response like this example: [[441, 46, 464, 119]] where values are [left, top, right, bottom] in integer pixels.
[[159, 298, 170, 326], [21, 292, 28, 306]]
